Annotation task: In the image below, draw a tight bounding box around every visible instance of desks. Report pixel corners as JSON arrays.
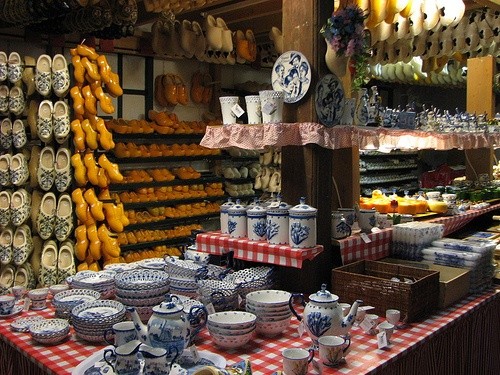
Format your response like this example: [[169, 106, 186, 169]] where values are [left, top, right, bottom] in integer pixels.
[[0, 283, 500, 375]]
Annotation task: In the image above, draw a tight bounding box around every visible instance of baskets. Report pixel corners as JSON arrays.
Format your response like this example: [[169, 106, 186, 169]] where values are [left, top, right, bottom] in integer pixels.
[[332, 259, 440, 324]]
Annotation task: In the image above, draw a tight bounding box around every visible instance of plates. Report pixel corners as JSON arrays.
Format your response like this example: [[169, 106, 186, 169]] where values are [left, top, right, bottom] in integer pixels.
[[271, 50, 346, 128], [0, 305, 24, 317]]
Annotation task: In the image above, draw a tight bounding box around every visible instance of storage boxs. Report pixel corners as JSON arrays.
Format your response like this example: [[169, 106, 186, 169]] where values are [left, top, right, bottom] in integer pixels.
[[379, 257, 469, 308]]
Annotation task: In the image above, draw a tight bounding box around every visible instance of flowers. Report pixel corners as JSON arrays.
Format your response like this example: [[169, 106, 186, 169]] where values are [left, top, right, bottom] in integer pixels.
[[322, 0, 372, 91]]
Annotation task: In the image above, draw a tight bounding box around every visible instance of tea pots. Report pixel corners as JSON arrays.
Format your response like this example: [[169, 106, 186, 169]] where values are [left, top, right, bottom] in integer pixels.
[[288, 283, 363, 349], [126, 293, 208, 359]]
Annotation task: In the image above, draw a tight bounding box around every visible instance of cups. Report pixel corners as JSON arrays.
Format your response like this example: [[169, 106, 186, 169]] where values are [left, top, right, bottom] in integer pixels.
[[220, 193, 317, 248], [340, 303, 350, 316], [353, 307, 365, 327], [13, 286, 25, 299], [332, 206, 393, 239], [317, 334, 351, 367], [281, 347, 314, 375], [340, 97, 356, 125], [219, 90, 285, 125], [32, 299, 47, 310], [104, 320, 136, 348]]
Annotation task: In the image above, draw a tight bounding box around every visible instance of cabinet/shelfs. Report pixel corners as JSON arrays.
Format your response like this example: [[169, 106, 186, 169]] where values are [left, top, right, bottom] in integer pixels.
[[106, 124, 500, 258]]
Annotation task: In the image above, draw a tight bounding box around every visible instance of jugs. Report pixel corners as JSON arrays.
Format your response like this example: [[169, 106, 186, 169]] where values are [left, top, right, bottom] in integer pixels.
[[103, 340, 143, 375], [139, 344, 179, 375]]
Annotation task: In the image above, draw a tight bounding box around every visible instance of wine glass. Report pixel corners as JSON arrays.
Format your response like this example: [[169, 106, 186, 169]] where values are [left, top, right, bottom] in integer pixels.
[[366, 315, 379, 335], [386, 309, 401, 330], [378, 323, 394, 344]]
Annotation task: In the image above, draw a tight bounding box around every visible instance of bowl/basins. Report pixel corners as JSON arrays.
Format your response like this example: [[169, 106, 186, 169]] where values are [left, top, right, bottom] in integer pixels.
[[27, 288, 48, 301], [49, 284, 68, 295], [0, 296, 15, 314], [208, 290, 293, 349]]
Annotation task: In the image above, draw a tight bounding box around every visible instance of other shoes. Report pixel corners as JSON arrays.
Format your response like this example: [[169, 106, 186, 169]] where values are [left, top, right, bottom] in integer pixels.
[[69, 44, 129, 271], [355, 0, 500, 85], [152, 15, 256, 64], [0, 52, 75, 295], [105, 110, 280, 264], [155, 72, 189, 108], [190, 71, 213, 103]]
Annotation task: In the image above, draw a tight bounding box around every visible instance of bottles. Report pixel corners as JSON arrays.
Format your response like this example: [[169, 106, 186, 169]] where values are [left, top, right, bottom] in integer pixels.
[[354, 95, 369, 126]]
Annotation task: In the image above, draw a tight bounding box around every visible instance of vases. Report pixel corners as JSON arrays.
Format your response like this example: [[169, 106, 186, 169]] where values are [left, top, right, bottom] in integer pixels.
[[320, 30, 347, 77]]
